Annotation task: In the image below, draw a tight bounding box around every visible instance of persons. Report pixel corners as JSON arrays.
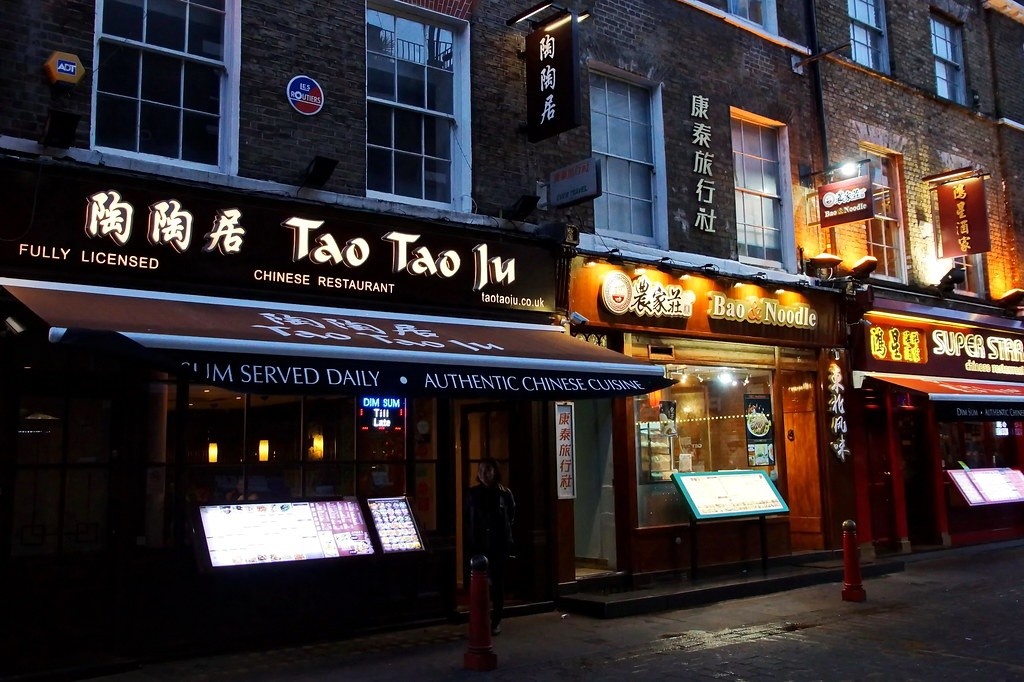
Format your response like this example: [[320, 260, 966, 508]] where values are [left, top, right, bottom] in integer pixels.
[[464, 458, 516, 636]]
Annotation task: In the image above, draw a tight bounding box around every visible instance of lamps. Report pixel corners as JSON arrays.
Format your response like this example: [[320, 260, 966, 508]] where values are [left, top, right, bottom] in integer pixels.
[[513, 195, 541, 219], [938, 268, 965, 291], [740, 374, 750, 387], [852, 256, 878, 274], [998, 288, 1024, 305], [305, 156, 339, 188], [38, 110, 82, 149], [807, 253, 843, 268], [697, 374, 704, 382]]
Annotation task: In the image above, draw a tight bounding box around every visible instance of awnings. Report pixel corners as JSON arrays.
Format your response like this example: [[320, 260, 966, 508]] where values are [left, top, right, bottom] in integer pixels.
[[0, 275, 679, 399], [851, 369, 1024, 423]]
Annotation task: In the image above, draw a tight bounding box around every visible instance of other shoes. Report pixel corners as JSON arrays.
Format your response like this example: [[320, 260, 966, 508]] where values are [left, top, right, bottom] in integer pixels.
[[491, 623, 502, 636]]
